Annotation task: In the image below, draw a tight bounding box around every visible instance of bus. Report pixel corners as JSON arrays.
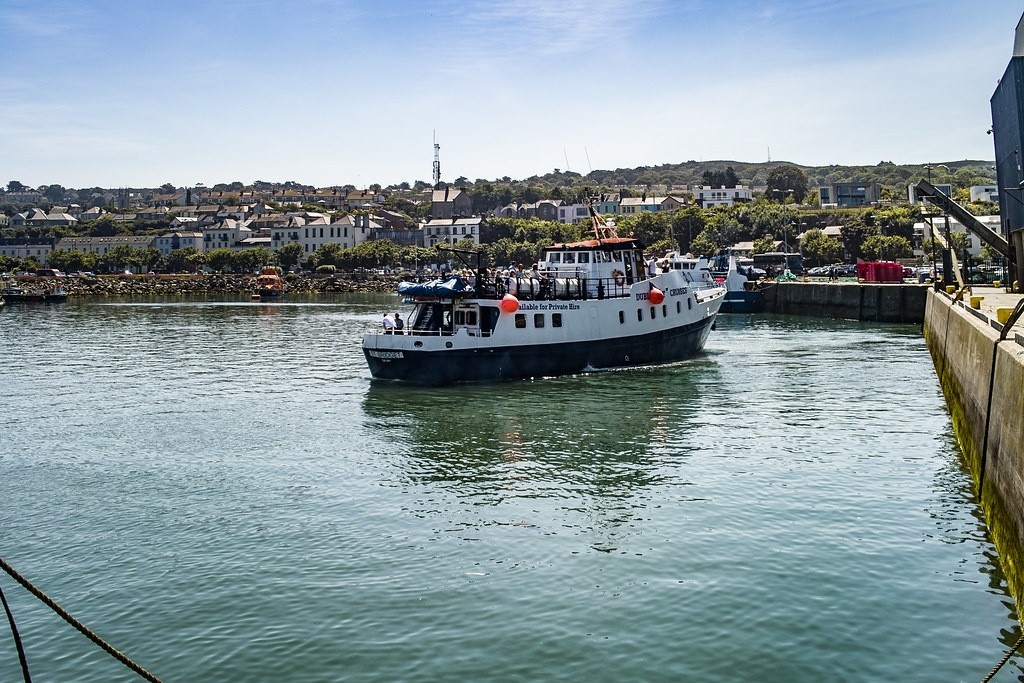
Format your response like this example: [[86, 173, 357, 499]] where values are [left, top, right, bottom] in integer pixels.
[[753, 252, 802, 275]]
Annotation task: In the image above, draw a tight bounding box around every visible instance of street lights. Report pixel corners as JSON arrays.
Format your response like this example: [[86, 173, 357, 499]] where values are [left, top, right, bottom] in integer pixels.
[[923, 164, 938, 283], [792, 223, 809, 253], [871, 216, 888, 263], [773, 189, 794, 270], [682, 204, 699, 253], [415, 219, 426, 270], [667, 223, 673, 250]]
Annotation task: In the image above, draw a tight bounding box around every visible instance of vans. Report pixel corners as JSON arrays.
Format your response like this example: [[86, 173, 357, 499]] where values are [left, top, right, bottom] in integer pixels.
[[36, 269, 63, 278], [118, 270, 132, 275]]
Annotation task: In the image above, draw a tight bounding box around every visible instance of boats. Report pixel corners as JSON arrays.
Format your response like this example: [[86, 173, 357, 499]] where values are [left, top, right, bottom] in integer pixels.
[[36, 285, 68, 301], [253, 259, 287, 298], [1, 287, 44, 302], [642, 250, 766, 308], [362, 144, 729, 384]]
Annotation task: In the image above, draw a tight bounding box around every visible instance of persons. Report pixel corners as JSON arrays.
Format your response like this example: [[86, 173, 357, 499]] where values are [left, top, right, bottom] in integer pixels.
[[383, 313, 404, 335], [662, 260, 670, 273], [829, 268, 838, 280], [431, 261, 547, 285], [748, 266, 754, 278], [648, 257, 658, 278], [447, 308, 456, 330], [765, 264, 775, 279]]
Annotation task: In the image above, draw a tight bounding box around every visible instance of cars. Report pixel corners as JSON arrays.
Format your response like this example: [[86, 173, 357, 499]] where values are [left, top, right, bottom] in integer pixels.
[[0, 272, 36, 279], [148, 268, 251, 275], [61, 272, 95, 277], [354, 267, 433, 274], [806, 262, 1008, 284], [94, 270, 114, 275]]
